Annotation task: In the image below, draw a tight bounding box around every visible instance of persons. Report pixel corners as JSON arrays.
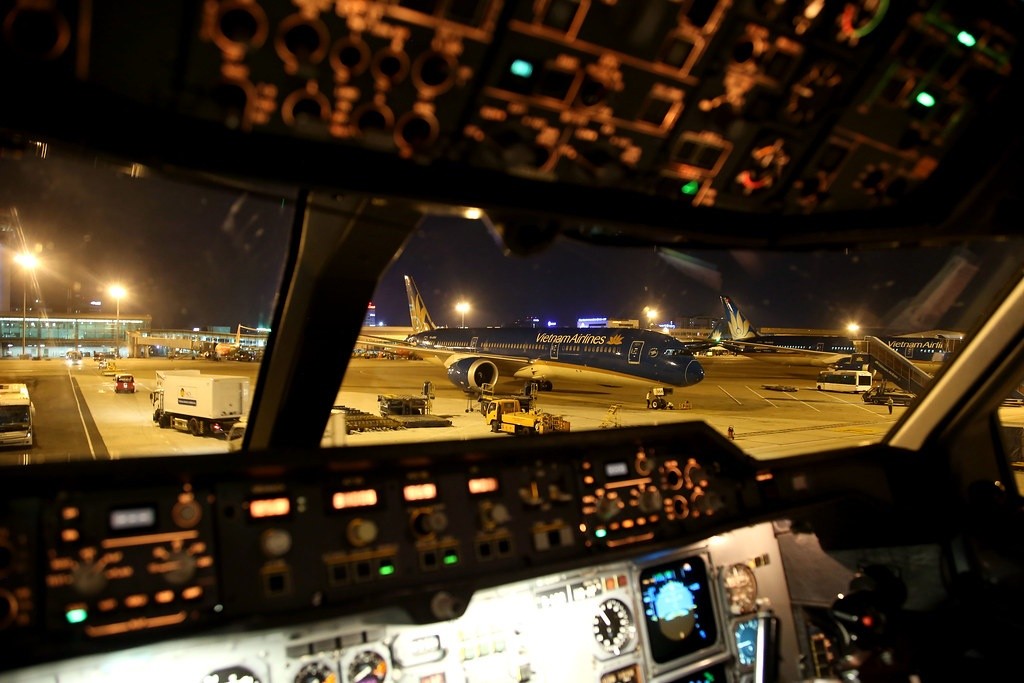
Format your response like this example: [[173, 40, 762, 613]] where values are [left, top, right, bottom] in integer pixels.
[[887, 396, 894, 415], [666, 401, 676, 410], [646, 391, 652, 409]]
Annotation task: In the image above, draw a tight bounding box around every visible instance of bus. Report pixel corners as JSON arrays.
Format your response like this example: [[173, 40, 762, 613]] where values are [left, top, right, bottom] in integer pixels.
[[816, 370, 872, 395]]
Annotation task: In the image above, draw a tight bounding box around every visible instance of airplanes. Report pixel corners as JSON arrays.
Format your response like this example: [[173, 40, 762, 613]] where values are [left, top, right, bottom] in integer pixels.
[[686, 292, 971, 405], [169, 323, 259, 362], [353, 273, 742, 413]]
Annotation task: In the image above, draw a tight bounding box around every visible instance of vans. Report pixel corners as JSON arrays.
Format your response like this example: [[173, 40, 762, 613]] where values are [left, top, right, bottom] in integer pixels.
[[66, 350, 82, 365]]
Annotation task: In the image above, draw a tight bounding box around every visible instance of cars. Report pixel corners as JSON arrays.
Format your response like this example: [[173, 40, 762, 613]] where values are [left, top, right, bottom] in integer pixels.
[[112, 374, 135, 394]]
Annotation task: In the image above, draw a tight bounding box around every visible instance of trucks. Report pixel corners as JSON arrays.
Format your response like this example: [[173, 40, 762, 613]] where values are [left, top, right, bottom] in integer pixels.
[[486, 399, 571, 438], [0, 383, 37, 448], [149, 368, 243, 437], [98, 362, 117, 376]]
